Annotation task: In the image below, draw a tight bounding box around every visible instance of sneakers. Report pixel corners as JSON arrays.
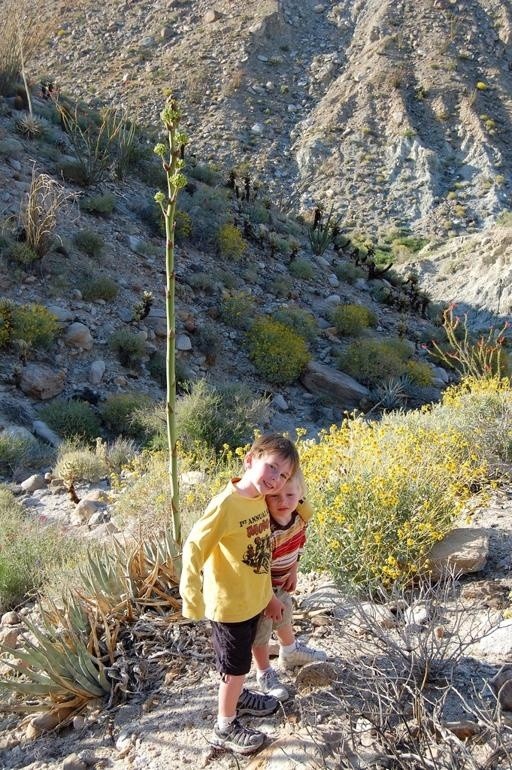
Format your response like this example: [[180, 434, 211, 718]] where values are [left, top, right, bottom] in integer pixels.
[[279, 643, 325, 669], [213, 719, 266, 754], [236, 690, 279, 718], [257, 671, 289, 700]]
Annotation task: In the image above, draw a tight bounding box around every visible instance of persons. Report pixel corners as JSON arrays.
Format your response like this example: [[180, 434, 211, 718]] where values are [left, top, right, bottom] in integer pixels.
[[178, 431, 314, 754], [250, 465, 330, 702]]
